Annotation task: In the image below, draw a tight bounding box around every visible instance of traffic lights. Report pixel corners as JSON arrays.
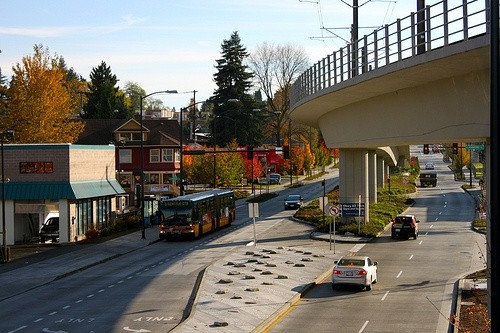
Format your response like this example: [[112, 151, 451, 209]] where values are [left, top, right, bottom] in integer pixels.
[[215, 175, 220, 180], [423, 144, 429, 154], [452, 142, 458, 154]]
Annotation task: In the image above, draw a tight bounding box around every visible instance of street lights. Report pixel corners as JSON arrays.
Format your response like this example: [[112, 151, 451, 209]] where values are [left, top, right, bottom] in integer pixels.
[[273, 111, 293, 185], [140, 90, 179, 239], [179, 99, 241, 195], [213, 108, 260, 188]]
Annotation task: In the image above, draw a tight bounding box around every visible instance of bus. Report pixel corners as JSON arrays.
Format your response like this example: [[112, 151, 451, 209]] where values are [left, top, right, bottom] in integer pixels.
[[155, 189, 236, 242]]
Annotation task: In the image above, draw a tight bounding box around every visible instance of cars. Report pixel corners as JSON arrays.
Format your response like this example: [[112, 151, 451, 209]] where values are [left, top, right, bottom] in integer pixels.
[[39, 217, 60, 244], [331, 256, 378, 291], [284, 194, 303, 210], [391, 215, 420, 240], [425, 162, 434, 170]]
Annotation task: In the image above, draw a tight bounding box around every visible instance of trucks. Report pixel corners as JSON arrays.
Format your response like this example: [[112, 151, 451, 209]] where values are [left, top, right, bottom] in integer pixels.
[[269, 173, 281, 185], [418, 170, 437, 187]]
